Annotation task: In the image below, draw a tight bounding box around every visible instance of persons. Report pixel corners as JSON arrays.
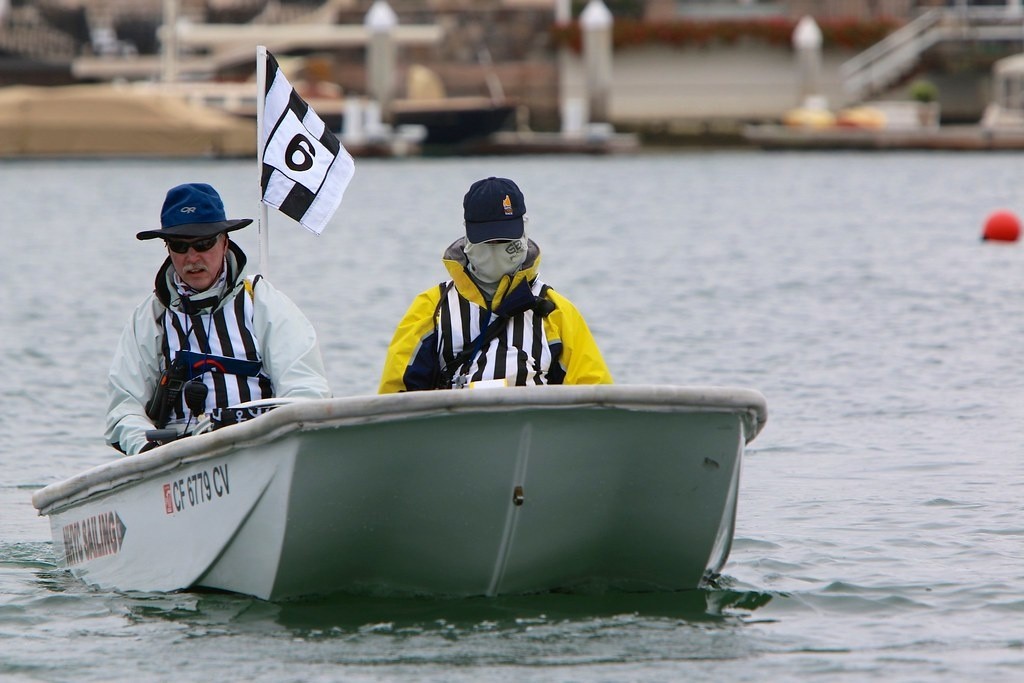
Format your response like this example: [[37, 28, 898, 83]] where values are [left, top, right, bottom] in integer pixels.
[[377, 176, 614, 394], [103, 182, 332, 456]]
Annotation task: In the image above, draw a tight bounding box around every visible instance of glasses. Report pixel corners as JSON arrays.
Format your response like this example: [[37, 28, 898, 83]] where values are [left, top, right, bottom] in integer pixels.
[[165, 232, 222, 254]]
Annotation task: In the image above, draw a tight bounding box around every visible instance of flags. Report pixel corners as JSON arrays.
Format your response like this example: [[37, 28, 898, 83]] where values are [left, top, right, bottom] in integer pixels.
[[260, 51, 355, 234]]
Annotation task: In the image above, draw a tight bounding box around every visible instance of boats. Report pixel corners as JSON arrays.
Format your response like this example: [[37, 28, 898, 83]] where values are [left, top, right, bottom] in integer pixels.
[[598, 110, 990, 149], [1, 26, 517, 156], [31, 382, 767, 610]]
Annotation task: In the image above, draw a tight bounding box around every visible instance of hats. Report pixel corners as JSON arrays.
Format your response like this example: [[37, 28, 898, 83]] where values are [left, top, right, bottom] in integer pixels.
[[136, 182, 254, 240], [463, 176, 526, 245]]
